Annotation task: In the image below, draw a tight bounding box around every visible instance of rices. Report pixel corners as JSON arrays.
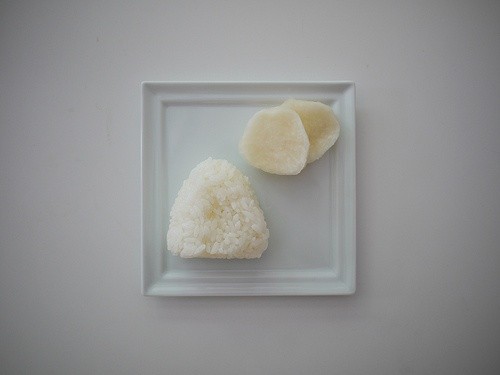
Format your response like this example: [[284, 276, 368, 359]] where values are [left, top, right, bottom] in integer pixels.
[[165, 156, 271, 262]]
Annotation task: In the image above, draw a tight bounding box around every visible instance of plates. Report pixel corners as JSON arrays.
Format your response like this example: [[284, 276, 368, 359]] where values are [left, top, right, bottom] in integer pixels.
[[140, 80, 356, 296]]
